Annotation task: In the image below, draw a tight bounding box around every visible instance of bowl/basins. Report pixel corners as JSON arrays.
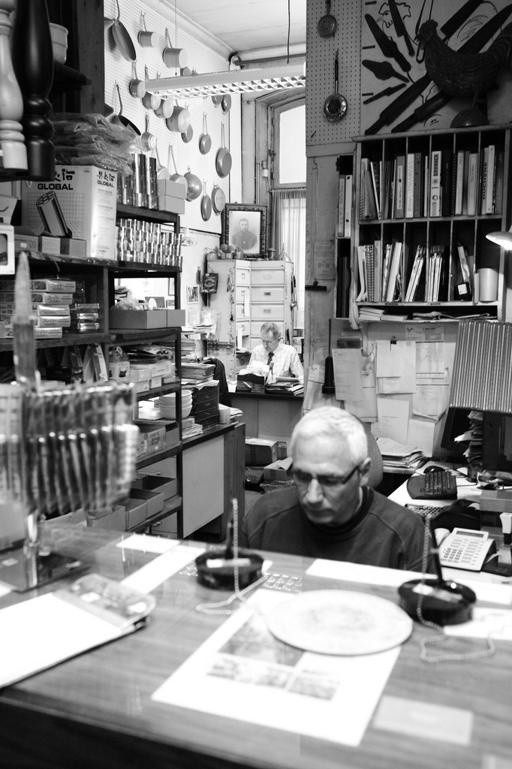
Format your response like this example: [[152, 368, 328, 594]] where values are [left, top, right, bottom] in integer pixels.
[[158, 389, 193, 420]]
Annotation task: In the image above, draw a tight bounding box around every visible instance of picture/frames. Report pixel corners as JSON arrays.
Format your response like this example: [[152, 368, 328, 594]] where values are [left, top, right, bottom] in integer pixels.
[[224, 203, 269, 259]]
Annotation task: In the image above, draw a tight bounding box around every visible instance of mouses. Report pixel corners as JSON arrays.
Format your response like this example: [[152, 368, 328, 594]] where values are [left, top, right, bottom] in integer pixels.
[[424, 466, 445, 473]]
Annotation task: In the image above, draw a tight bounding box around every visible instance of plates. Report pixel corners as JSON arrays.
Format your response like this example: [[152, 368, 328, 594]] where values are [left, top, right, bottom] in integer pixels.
[[263, 588, 412, 656]]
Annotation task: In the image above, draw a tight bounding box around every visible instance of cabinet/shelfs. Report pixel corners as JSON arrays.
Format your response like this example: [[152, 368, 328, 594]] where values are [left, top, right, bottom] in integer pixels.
[[351, 123, 511, 321], [0, 173, 184, 541], [206, 259, 252, 350], [249, 261, 291, 354]]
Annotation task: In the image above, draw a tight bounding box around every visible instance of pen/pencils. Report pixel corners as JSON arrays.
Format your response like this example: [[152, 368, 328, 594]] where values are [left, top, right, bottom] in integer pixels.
[[226, 497, 249, 558], [26, 505, 42, 542], [426, 512, 470, 580]]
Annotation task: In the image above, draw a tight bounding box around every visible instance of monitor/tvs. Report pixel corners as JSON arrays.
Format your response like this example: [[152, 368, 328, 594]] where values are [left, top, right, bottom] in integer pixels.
[[441, 406, 499, 470]]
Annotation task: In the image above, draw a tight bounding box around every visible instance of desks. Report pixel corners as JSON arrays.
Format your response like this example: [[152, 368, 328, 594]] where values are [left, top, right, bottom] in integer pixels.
[[228, 382, 305, 442], [386, 458, 512, 582], [137, 420, 246, 543]]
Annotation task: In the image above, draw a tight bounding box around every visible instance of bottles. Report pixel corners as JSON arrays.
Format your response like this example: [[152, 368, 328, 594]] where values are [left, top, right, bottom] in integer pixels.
[[206, 243, 278, 261]]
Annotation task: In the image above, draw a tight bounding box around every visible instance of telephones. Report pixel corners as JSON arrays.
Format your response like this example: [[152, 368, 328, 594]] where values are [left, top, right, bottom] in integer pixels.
[[434, 527, 497, 573]]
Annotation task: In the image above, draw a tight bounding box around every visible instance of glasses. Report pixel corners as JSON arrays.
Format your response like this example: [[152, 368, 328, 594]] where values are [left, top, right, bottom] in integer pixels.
[[285, 460, 360, 489]]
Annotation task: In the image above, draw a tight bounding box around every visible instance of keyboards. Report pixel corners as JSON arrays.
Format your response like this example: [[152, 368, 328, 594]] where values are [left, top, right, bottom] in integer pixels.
[[407, 471, 457, 500]]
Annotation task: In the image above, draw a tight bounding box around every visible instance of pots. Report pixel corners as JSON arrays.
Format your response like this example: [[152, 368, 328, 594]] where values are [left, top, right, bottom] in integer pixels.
[[113, 0, 237, 220]]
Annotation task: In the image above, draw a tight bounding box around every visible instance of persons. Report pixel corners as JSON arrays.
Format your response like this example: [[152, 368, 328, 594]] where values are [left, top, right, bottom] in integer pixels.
[[249, 322, 304, 383], [240, 405, 439, 577], [231, 220, 257, 250]]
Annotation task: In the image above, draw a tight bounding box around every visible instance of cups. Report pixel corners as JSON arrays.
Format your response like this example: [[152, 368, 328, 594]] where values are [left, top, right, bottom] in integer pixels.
[[182, 301, 212, 329], [118, 219, 182, 267], [49, 22, 69, 65]]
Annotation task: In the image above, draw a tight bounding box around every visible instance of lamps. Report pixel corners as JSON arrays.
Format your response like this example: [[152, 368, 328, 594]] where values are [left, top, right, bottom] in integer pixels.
[[144, 0, 306, 101], [448, 320, 512, 483]]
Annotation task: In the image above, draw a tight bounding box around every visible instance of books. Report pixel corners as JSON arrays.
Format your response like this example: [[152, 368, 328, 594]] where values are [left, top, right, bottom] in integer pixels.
[[357, 144, 495, 303]]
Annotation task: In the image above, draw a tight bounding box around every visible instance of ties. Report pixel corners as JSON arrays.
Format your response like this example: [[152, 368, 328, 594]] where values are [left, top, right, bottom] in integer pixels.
[[266, 351, 275, 366]]
